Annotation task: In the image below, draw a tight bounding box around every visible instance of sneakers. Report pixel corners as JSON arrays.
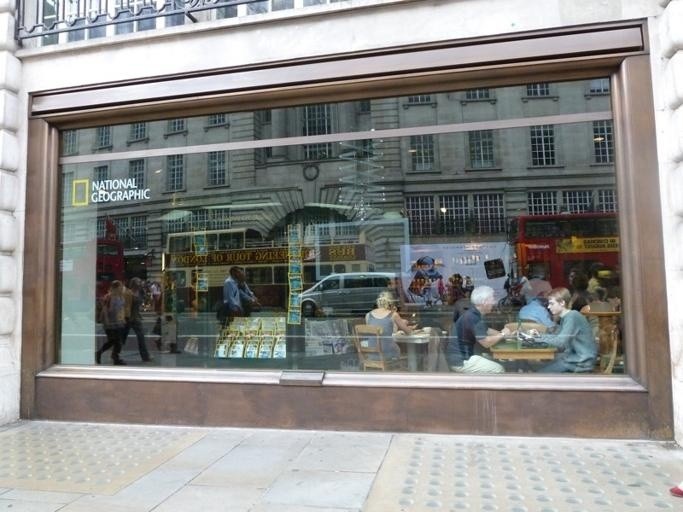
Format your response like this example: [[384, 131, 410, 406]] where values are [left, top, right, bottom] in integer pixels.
[[96, 351, 101, 364]]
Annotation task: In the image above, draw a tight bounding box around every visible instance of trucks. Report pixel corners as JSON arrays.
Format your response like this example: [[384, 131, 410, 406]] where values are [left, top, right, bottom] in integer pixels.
[[397, 233, 519, 325]]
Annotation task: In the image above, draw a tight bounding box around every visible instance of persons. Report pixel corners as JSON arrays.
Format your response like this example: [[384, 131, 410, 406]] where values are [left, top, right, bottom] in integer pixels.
[[125, 277, 155, 361], [434, 275, 445, 305], [223, 266, 255, 317], [586, 262, 606, 293], [524, 286, 597, 373], [365, 291, 411, 361], [518, 280, 559, 334], [96, 280, 129, 365], [446, 285, 511, 373], [580, 287, 619, 353], [568, 268, 589, 297]]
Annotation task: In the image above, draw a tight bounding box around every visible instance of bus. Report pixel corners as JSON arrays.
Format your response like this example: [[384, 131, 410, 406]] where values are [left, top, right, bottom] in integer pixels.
[[159, 218, 374, 316], [58, 239, 124, 324], [508, 211, 620, 309]]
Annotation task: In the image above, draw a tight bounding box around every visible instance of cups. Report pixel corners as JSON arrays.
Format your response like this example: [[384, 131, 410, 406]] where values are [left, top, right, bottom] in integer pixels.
[[422, 327, 432, 334]]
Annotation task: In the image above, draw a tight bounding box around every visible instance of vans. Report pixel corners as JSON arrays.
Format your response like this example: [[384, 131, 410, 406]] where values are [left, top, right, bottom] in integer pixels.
[[292, 268, 413, 322]]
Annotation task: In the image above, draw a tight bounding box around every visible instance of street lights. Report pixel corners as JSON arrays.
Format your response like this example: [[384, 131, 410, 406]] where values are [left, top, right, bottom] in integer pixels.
[[440, 207, 448, 240]]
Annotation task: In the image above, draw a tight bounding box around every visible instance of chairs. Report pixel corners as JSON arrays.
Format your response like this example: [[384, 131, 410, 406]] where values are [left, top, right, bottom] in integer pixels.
[[351, 311, 625, 373]]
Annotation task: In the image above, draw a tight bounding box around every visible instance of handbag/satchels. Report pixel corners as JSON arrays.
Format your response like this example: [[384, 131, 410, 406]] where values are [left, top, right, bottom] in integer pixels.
[[216, 302, 234, 322], [96, 292, 111, 323]]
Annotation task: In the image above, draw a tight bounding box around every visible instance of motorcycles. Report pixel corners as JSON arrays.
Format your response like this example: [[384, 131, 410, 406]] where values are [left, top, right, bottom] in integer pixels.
[[494, 276, 541, 313]]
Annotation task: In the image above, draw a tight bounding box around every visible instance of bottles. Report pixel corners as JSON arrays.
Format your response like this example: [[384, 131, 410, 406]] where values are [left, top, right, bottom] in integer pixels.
[[408, 279, 441, 294], [452, 271, 485, 288], [451, 254, 487, 265], [410, 312, 418, 332], [516, 319, 522, 340], [504, 320, 511, 341]]
